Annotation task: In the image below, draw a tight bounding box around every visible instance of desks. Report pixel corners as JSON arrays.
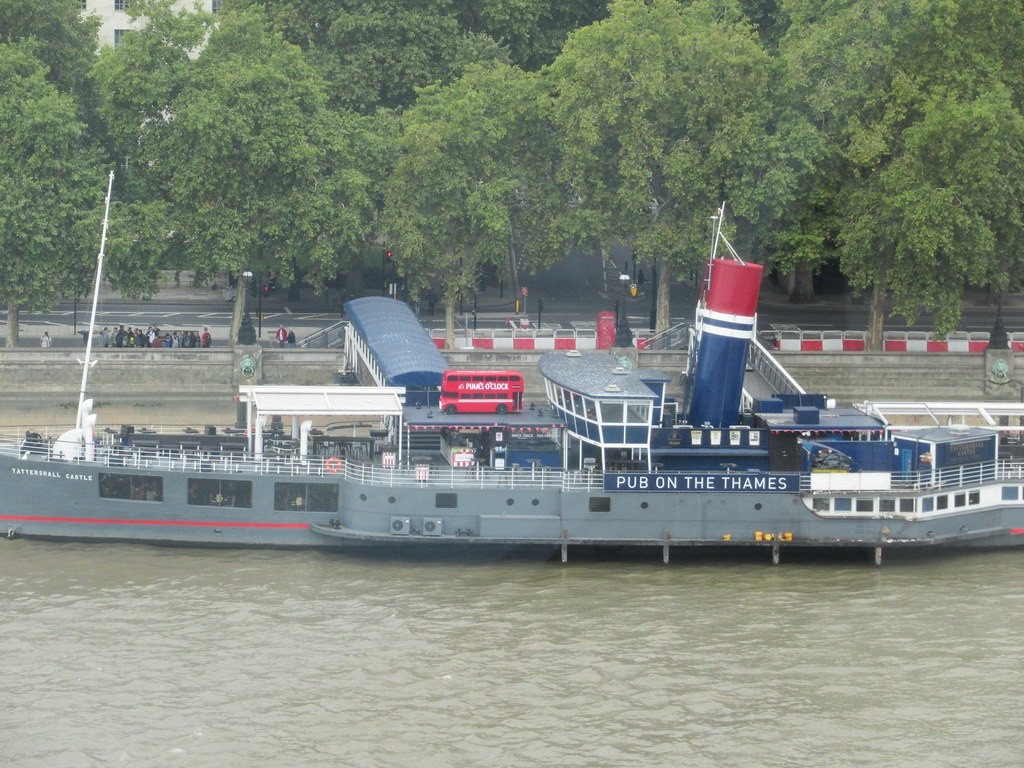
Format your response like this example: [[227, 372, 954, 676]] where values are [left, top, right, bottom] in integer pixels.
[[600, 460, 647, 473], [381, 444, 398, 469], [370, 430, 388, 453], [313, 437, 374, 460], [470, 458, 486, 462], [132, 440, 159, 456], [179, 441, 200, 458], [583, 462, 601, 485], [720, 463, 736, 474], [412, 456, 433, 481], [220, 442, 247, 460], [651, 463, 664, 473], [525, 458, 541, 463]]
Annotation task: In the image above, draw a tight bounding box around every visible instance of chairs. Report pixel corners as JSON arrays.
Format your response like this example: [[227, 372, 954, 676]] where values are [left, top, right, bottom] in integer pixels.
[[262, 436, 368, 460]]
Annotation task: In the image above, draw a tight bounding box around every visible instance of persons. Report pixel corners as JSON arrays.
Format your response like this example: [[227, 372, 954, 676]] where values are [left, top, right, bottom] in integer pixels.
[[286, 328, 297, 348], [174, 270, 181, 288], [96, 324, 212, 348], [40, 331, 52, 348], [275, 324, 288, 348]]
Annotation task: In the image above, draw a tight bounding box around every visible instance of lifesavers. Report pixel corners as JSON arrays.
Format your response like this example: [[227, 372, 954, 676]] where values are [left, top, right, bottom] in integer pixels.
[[325, 457, 342, 474], [243, 427, 257, 442]]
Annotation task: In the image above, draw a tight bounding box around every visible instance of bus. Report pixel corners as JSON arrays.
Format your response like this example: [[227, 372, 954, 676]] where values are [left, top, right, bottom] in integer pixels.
[[438, 370, 525, 415]]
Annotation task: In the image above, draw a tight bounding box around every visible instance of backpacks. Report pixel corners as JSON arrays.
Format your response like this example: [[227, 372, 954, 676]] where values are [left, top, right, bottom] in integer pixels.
[[206, 334, 211, 346]]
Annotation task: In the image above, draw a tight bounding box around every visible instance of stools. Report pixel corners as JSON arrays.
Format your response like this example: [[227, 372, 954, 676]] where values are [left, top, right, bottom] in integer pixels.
[[465, 466, 489, 480], [573, 466, 630, 486], [498, 464, 551, 486]]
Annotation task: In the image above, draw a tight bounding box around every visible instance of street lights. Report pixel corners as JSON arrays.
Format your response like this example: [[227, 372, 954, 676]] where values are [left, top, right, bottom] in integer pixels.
[[616, 260, 636, 345], [238, 270, 258, 344]]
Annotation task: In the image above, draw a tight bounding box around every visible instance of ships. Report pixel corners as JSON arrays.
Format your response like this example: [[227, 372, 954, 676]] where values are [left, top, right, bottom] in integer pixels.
[[1, 160, 1024, 567]]
